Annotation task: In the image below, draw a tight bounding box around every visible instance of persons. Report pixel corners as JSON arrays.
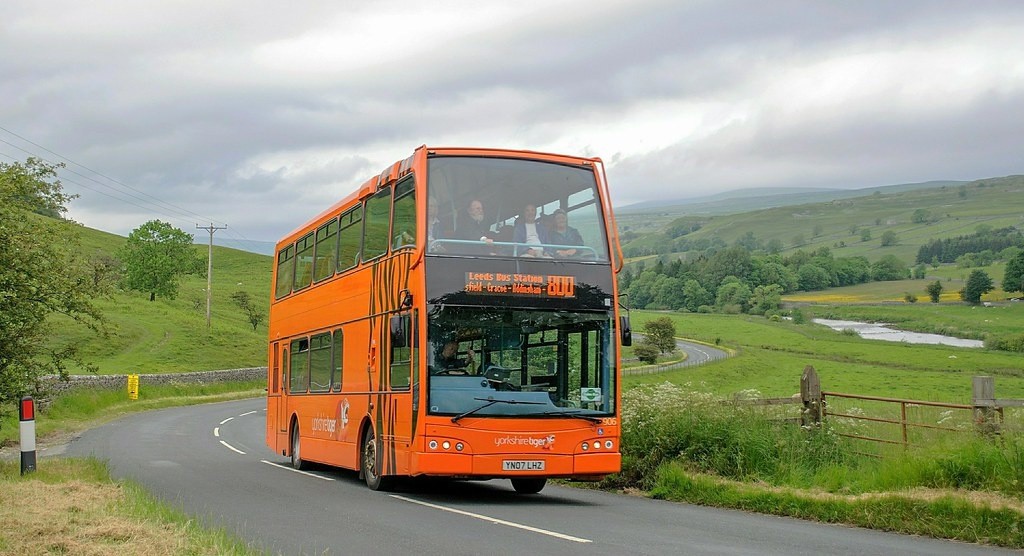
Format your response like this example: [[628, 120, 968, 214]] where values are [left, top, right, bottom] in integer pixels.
[[424, 196, 448, 241], [458, 197, 496, 257], [547, 208, 586, 262], [432, 340, 477, 380], [509, 202, 555, 260]]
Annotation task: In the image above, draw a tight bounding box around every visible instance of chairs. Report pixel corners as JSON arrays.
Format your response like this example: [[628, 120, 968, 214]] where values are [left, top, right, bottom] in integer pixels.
[[276, 226, 515, 298]]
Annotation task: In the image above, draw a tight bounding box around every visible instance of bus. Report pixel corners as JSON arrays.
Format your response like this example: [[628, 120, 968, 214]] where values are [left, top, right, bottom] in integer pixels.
[[263, 144, 624, 497]]
